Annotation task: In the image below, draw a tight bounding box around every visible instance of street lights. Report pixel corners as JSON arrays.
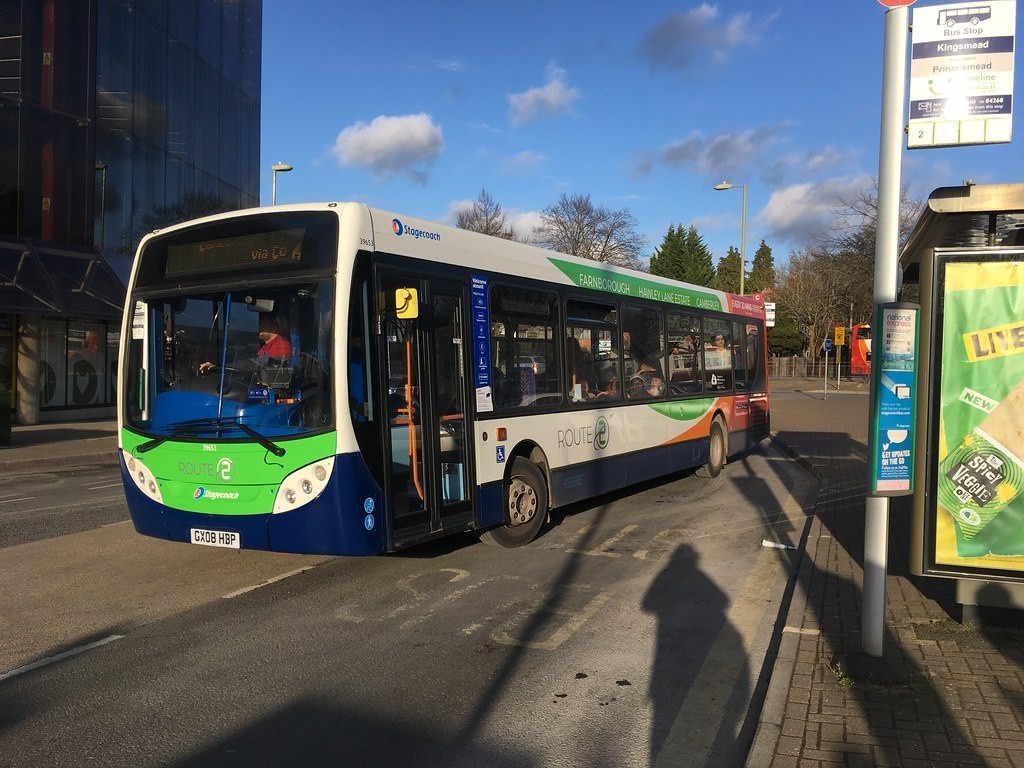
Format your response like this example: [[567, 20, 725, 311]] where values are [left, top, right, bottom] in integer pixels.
[[273, 165, 294, 206], [714, 183, 747, 297], [95, 162, 107, 255]]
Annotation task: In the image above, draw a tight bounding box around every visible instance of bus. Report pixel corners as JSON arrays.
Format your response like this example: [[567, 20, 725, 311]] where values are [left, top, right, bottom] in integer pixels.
[[850, 324, 872, 374], [116, 201, 772, 557]]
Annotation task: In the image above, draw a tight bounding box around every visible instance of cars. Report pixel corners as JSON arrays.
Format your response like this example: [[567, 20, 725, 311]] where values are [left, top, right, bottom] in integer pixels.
[[497, 356, 546, 379]]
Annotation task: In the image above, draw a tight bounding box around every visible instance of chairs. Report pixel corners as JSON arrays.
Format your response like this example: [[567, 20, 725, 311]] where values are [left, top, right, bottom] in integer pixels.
[[493, 350, 745, 409]]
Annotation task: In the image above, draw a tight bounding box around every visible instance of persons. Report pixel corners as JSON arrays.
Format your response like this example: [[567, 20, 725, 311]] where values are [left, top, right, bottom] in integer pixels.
[[711, 332, 725, 347], [629, 375, 646, 398], [579, 377, 596, 399], [200, 314, 291, 374]]
